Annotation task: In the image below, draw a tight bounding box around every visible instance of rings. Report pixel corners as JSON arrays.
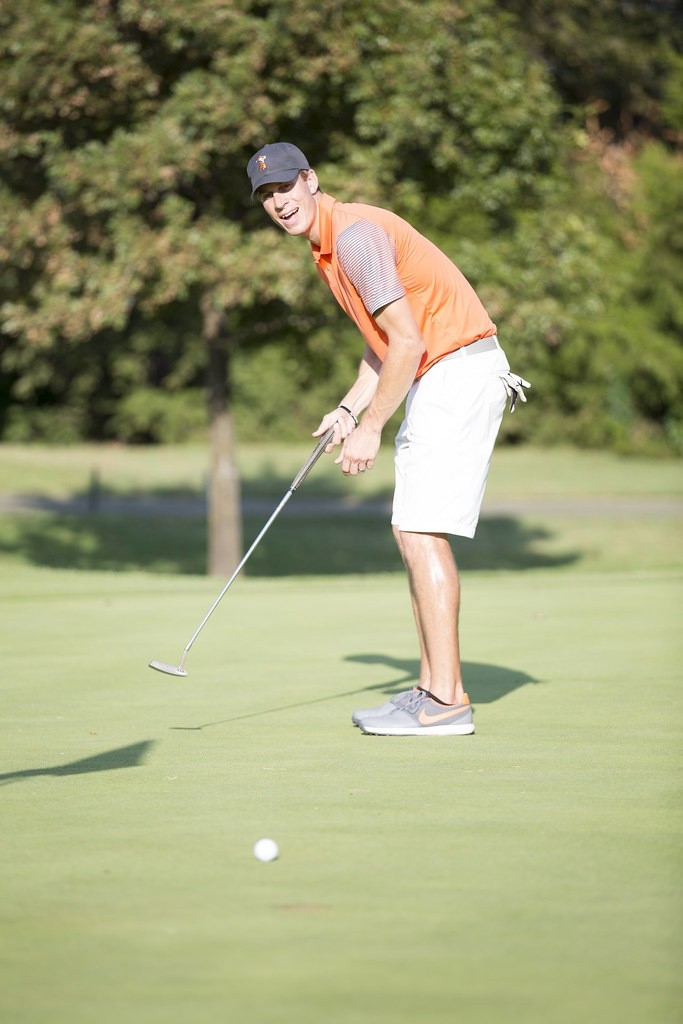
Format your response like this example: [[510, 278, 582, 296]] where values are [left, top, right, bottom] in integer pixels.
[[358, 468, 365, 472]]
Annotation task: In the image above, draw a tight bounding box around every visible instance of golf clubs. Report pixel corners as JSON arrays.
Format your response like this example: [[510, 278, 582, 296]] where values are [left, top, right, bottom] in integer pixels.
[[147, 426, 336, 677]]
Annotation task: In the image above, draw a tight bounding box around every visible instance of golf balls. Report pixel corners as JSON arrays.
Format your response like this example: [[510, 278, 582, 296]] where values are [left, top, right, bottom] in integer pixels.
[[254, 838, 279, 864]]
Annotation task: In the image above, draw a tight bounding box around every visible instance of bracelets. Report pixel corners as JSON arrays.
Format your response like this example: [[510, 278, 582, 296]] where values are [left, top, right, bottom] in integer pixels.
[[337, 405, 359, 429]]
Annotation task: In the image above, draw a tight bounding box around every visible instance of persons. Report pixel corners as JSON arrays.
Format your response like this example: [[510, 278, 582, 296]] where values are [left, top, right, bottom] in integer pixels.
[[248, 144, 531, 736]]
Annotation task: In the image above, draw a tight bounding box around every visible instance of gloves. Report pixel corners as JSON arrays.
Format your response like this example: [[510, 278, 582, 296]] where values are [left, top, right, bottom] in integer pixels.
[[500, 371, 531, 413]]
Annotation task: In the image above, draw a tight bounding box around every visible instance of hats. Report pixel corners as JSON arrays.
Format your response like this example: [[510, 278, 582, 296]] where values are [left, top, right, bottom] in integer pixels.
[[247, 142, 310, 202]]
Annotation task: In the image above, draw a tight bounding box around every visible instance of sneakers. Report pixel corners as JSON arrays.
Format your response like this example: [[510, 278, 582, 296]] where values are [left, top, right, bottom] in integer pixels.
[[359, 691, 475, 736], [352, 686, 428, 725]]
